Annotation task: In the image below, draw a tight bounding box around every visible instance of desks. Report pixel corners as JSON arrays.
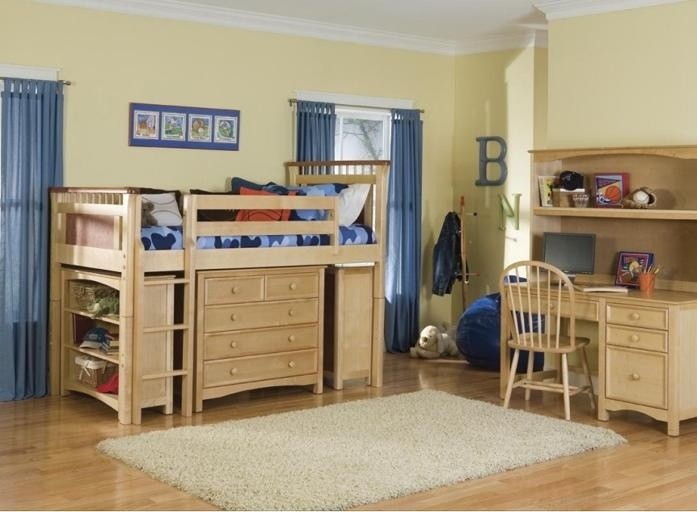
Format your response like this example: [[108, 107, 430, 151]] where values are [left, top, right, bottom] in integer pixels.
[[500, 279, 696, 436]]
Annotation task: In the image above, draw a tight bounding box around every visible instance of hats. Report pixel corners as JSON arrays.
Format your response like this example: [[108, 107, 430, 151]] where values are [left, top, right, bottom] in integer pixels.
[[554, 170, 582, 191], [79, 327, 109, 350], [96, 374, 118, 395]]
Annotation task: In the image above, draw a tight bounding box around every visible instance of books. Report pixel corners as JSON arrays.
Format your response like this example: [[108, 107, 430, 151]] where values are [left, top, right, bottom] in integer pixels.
[[574, 282, 629, 293], [102, 332, 120, 356]]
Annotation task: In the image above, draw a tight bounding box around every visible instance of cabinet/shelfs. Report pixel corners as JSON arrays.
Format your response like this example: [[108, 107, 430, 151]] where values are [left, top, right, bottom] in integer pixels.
[[192, 266, 328, 413], [61, 267, 176, 425], [323, 264, 374, 390], [527, 144, 696, 295]]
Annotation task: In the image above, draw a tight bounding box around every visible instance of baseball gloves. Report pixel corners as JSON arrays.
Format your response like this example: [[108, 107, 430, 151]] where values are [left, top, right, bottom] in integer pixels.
[[623, 187, 658, 209]]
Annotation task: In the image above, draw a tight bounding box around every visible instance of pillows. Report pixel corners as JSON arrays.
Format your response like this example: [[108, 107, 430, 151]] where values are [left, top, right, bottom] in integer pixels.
[[132, 177, 371, 230]]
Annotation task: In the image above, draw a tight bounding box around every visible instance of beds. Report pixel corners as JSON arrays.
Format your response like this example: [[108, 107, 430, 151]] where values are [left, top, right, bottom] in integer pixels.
[[48, 158, 391, 426]]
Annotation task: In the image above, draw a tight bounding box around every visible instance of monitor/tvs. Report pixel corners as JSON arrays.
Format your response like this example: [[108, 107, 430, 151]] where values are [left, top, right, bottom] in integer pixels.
[[541, 232, 595, 288]]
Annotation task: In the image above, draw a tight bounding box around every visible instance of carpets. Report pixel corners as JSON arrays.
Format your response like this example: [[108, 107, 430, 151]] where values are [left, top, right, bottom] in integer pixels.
[[93, 388, 628, 510]]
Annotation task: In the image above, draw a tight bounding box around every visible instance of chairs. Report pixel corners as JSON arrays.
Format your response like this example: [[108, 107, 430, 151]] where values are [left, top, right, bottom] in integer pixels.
[[499, 260, 595, 421]]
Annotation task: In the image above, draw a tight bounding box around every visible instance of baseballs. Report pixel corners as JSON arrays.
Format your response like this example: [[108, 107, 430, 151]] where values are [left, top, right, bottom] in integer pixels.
[[632, 191, 650, 204]]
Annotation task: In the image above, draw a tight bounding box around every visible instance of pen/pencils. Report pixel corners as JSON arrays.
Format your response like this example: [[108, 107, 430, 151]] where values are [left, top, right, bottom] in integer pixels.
[[643, 263, 661, 274]]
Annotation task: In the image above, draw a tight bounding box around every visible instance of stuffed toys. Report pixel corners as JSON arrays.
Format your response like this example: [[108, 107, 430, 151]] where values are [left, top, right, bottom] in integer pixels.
[[409, 327, 459, 359]]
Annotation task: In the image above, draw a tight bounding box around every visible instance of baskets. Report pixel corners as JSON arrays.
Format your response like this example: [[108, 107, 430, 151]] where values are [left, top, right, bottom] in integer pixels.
[[551, 187, 586, 207], [72, 284, 118, 311], [74, 354, 115, 387]]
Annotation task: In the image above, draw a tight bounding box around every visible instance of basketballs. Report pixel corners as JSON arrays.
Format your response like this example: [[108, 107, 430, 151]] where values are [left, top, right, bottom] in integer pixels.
[[606, 187, 622, 204]]
